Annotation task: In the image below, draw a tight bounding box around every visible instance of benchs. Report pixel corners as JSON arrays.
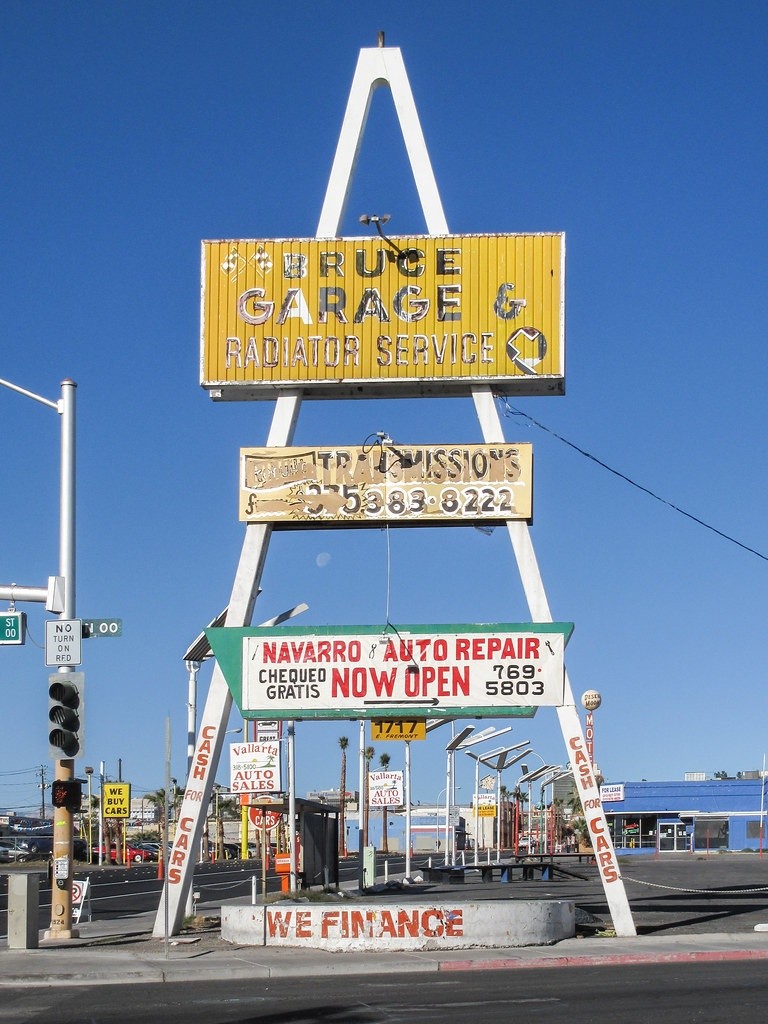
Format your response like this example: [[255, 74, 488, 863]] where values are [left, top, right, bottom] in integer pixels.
[[510, 853, 595, 864]]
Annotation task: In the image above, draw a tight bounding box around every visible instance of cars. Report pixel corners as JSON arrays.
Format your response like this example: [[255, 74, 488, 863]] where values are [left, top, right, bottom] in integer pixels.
[[518, 836, 535, 850], [143, 843, 173, 853], [235, 842, 285, 857], [28, 832, 86, 854], [222, 843, 252, 859], [87, 841, 148, 863], [136, 843, 170, 860], [11, 838, 31, 849], [0, 840, 35, 863], [203, 840, 235, 859]]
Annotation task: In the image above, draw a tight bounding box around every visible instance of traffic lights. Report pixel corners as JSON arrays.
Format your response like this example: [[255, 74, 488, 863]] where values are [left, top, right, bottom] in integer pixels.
[[52, 780, 75, 808], [47, 671, 84, 758]]
